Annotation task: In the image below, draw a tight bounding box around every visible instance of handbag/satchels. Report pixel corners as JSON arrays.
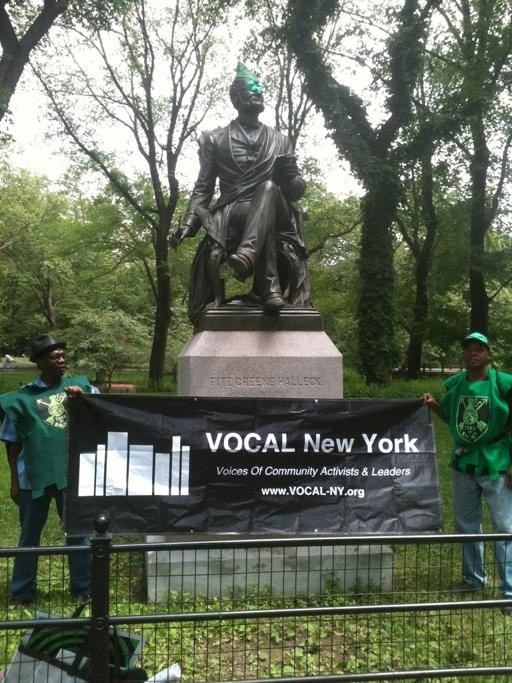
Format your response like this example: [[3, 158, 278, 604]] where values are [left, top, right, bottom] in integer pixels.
[[4, 636, 132, 682]]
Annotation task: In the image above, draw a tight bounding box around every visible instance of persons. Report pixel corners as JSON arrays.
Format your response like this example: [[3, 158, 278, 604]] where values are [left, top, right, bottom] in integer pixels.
[[0, 332, 101, 607], [417, 330, 511, 618], [169, 73, 313, 313]]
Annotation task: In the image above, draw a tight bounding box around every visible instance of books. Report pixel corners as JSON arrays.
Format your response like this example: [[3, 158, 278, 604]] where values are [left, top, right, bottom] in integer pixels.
[[273, 154, 297, 182]]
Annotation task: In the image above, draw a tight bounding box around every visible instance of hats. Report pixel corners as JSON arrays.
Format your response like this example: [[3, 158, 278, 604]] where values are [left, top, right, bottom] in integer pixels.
[[29, 335, 67, 363], [460, 332, 492, 351]]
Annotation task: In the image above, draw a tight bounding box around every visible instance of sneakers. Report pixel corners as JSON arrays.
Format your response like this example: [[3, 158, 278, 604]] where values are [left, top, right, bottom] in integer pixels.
[[6, 597, 27, 611], [72, 592, 92, 605], [452, 580, 477, 591], [500, 596, 511, 615]]
